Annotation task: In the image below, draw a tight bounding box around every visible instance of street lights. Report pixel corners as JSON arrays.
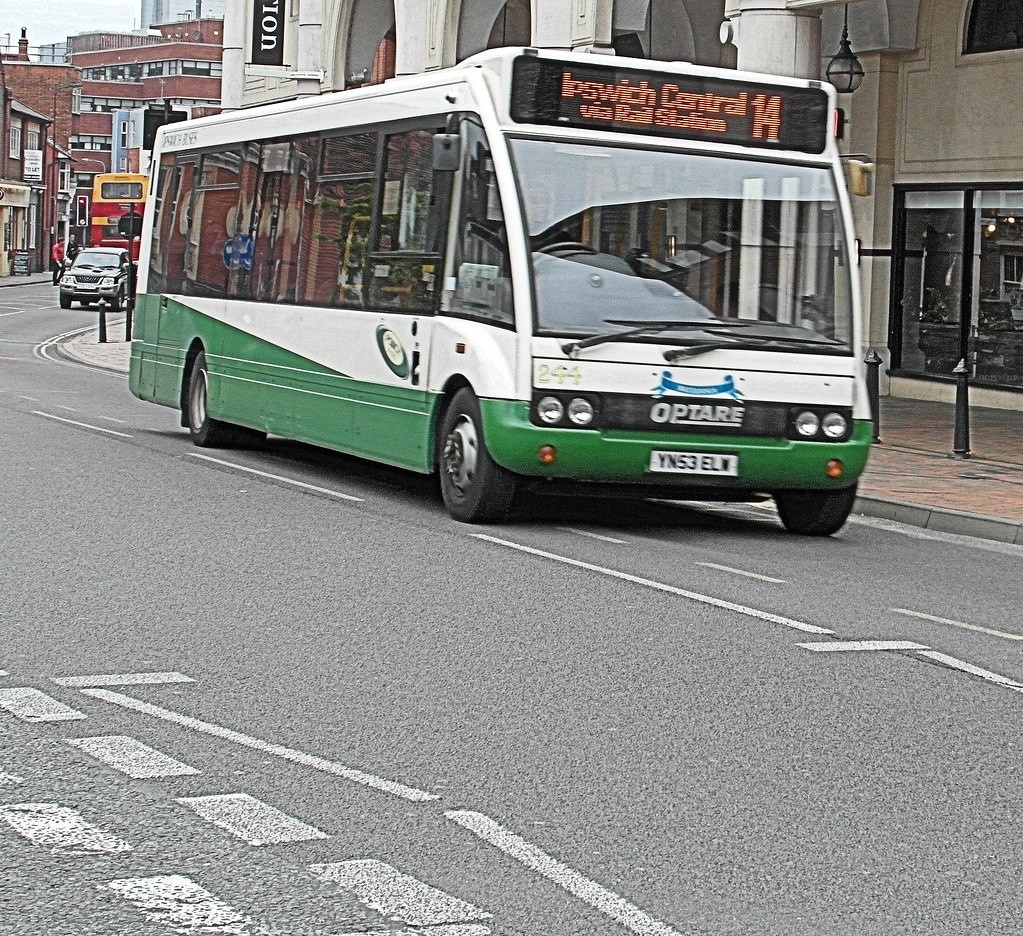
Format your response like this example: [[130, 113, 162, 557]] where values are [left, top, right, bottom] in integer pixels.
[[48, 84, 83, 271], [82, 158, 106, 174]]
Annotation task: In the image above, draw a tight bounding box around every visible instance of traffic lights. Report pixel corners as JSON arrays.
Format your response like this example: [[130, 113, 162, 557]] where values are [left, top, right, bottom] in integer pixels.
[[76, 195, 89, 227]]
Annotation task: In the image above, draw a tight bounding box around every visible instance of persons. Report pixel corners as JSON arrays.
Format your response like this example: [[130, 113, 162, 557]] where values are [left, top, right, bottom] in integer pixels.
[[51, 237, 66, 286], [66, 234, 80, 267]]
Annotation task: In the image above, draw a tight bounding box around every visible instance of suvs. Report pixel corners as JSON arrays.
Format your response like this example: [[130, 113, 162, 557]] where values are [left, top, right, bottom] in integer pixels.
[[58, 247, 132, 311]]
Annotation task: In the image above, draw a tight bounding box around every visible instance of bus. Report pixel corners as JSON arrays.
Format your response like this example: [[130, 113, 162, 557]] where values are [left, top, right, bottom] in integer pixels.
[[128, 44, 879, 538], [90, 172, 152, 261]]
